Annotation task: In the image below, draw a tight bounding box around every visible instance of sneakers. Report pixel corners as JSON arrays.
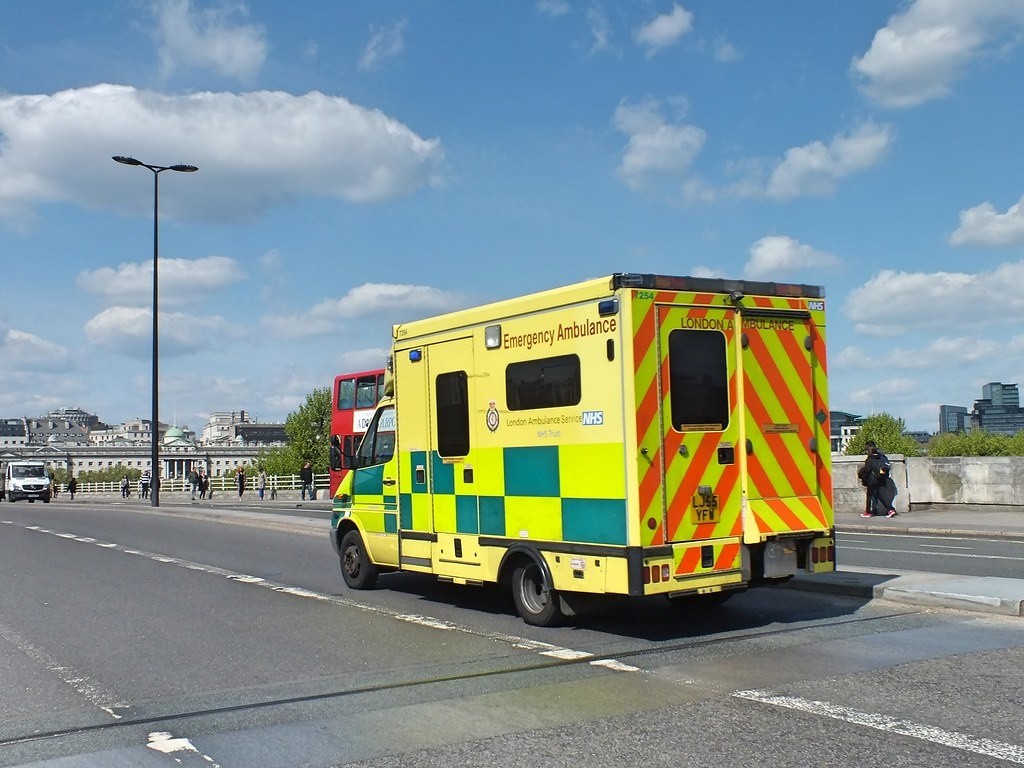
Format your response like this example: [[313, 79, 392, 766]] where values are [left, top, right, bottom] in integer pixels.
[[860, 512, 871, 518], [886, 510, 896, 517]]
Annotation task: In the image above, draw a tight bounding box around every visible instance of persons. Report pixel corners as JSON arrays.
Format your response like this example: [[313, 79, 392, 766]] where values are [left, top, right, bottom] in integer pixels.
[[66, 478, 77, 500], [300, 460, 317, 501], [197, 470, 209, 500], [235, 466, 246, 502], [861, 441, 895, 519], [121, 474, 130, 500], [139, 471, 150, 499], [187, 467, 199, 501], [255, 466, 268, 501], [52, 481, 58, 498]]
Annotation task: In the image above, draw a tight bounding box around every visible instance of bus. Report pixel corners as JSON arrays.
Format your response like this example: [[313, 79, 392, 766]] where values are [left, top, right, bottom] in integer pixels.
[[329, 367, 395, 500]]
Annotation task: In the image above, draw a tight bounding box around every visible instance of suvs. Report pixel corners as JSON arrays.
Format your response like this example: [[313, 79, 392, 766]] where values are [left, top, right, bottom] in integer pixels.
[[2, 461, 55, 503]]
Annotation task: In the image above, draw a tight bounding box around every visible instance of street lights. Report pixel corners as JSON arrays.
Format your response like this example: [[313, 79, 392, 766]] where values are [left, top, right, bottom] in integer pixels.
[[112, 155, 199, 507]]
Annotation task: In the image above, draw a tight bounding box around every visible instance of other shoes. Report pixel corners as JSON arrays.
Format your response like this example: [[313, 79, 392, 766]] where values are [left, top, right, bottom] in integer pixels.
[[239, 498, 242, 501], [871, 508, 878, 516], [123, 496, 131, 500]]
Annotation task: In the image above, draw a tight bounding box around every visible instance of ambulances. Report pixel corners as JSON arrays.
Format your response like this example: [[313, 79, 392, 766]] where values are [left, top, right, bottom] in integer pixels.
[[329, 272, 837, 626]]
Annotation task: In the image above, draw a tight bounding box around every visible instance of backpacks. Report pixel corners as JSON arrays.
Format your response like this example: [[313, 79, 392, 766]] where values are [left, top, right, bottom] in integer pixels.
[[871, 457, 889, 481]]
